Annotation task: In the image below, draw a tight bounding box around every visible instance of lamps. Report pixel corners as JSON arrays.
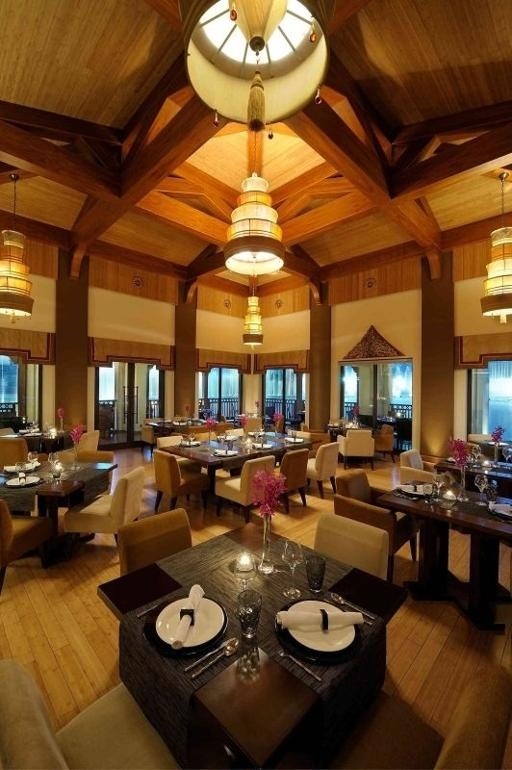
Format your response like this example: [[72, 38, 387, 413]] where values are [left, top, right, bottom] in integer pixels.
[[240, 274, 265, 346], [479, 166, 512, 328], [0, 168, 38, 324], [220, 0, 291, 277]]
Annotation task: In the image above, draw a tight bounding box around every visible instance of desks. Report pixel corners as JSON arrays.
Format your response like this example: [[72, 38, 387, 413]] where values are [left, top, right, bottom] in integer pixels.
[[383, 478, 512, 632], [1, 459, 119, 562], [98, 522, 408, 770]]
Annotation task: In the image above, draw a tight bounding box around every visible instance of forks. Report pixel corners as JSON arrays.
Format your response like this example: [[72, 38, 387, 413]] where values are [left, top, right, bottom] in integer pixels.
[[273, 642, 323, 683], [390, 490, 418, 503]]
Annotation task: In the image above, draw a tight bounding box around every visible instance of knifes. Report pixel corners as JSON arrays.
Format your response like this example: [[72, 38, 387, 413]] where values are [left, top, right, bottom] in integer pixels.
[[323, 598, 373, 627], [182, 637, 236, 671]]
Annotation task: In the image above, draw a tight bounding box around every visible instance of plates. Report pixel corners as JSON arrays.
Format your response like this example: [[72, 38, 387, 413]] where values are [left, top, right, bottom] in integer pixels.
[[6, 475, 41, 486], [490, 503, 512, 517], [145, 598, 230, 657], [276, 600, 360, 665]]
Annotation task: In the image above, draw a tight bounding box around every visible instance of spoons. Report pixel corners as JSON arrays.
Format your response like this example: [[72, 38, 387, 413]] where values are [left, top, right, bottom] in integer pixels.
[[324, 591, 378, 623], [192, 639, 239, 680]]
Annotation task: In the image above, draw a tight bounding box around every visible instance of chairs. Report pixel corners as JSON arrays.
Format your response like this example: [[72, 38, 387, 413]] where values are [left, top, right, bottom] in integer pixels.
[[1, 499, 54, 586], [312, 514, 391, 582], [333, 469, 418, 575], [68, 466, 143, 559], [1, 406, 511, 491], [116, 510, 192, 574], [212, 456, 275, 524]]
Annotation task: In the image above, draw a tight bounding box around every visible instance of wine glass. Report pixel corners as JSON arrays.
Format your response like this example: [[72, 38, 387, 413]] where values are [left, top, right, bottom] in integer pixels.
[[473, 474, 489, 507], [279, 539, 307, 600], [502, 447, 512, 469], [233, 553, 257, 588], [431, 473, 446, 500], [471, 446, 482, 468]]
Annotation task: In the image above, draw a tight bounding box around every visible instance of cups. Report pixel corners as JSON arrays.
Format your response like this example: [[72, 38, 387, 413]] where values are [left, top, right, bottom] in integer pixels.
[[17, 462, 27, 483], [237, 589, 263, 639], [28, 451, 61, 471], [26, 422, 56, 439], [186, 426, 298, 454], [235, 643, 261, 688], [304, 554, 326, 592]]
